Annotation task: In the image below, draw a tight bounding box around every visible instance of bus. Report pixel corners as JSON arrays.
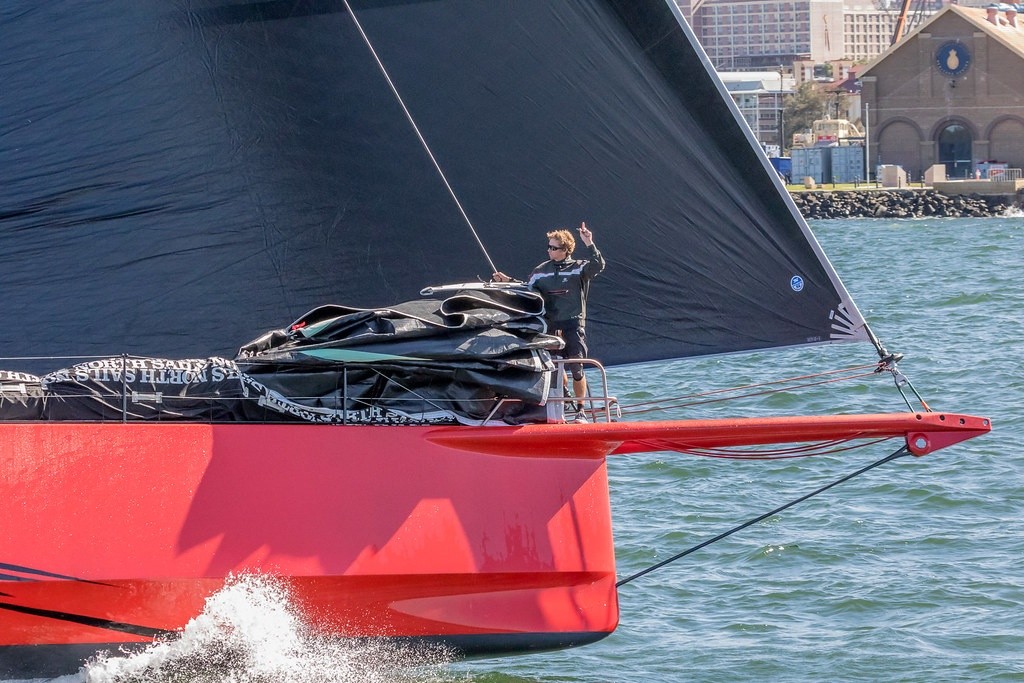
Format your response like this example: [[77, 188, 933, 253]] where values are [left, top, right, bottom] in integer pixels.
[[813, 119, 849, 145]]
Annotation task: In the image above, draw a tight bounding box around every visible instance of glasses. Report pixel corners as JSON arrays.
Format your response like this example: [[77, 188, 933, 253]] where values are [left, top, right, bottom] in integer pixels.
[[548, 245, 562, 251]]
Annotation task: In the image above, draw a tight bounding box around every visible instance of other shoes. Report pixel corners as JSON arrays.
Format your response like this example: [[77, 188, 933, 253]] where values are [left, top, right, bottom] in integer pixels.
[[575, 411, 588, 423], [564, 403, 571, 409]]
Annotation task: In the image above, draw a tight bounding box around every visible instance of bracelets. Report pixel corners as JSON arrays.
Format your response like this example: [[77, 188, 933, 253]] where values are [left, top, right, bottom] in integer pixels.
[[509, 277, 513, 282]]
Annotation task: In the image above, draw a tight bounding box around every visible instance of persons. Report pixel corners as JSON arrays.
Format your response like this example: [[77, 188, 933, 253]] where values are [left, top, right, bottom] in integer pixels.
[[493, 222, 605, 424]]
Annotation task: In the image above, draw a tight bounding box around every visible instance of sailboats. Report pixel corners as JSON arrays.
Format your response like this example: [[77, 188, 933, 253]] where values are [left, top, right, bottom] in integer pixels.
[[0, 0, 993, 680]]
